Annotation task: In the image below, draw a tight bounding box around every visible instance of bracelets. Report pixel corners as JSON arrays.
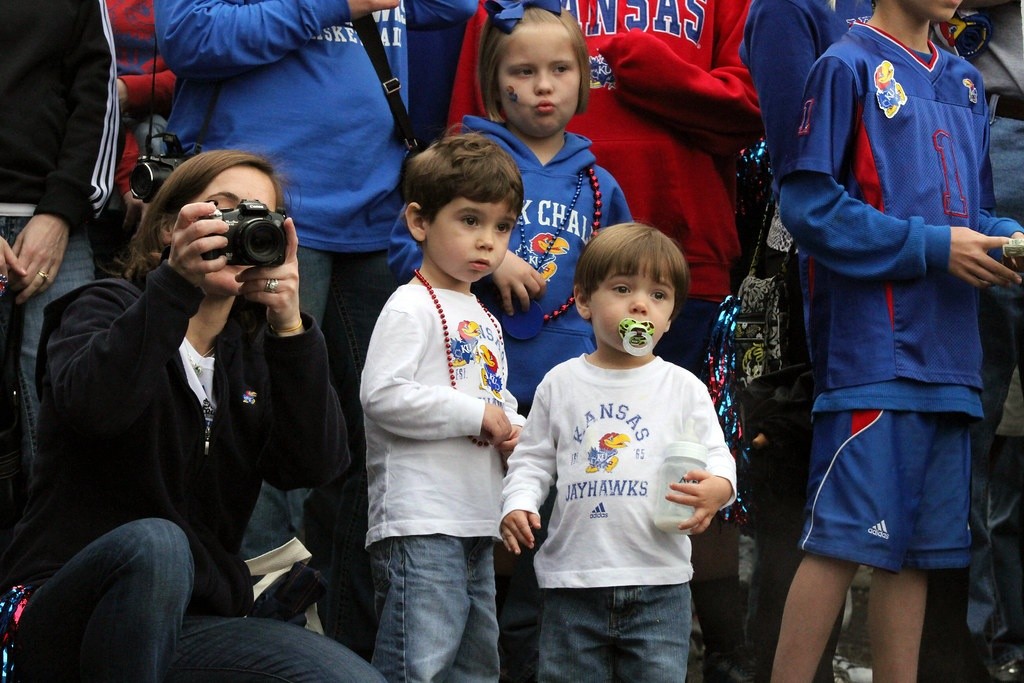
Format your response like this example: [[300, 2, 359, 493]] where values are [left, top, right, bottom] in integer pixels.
[[271, 320, 302, 332]]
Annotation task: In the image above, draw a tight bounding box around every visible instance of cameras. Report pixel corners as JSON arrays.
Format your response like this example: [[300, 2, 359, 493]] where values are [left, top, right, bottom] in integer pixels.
[[130, 133, 193, 203], [199, 198, 288, 266]]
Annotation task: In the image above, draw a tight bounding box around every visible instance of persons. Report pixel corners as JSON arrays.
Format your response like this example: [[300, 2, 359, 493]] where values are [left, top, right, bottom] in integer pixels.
[[0, 0, 1024, 683]]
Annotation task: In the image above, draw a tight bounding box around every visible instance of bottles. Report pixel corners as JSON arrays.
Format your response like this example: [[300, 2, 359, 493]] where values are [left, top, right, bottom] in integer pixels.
[[652, 445, 709, 536]]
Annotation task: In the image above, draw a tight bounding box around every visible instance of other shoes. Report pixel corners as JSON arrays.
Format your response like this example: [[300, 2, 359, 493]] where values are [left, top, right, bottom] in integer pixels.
[[702, 648, 759, 683]]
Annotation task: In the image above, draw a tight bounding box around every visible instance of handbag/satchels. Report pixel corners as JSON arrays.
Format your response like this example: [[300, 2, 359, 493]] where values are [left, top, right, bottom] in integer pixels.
[[730, 261, 815, 456]]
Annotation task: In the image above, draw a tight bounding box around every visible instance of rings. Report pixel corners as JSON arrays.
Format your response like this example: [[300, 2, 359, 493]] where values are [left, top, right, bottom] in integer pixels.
[[37, 271, 49, 280], [266, 278, 278, 291], [0, 274, 8, 297]]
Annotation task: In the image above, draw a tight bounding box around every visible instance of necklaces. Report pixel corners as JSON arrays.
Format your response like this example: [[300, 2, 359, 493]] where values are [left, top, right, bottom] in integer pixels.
[[184, 342, 214, 376], [540, 168, 602, 325], [502, 170, 583, 339], [415, 268, 506, 447]]
